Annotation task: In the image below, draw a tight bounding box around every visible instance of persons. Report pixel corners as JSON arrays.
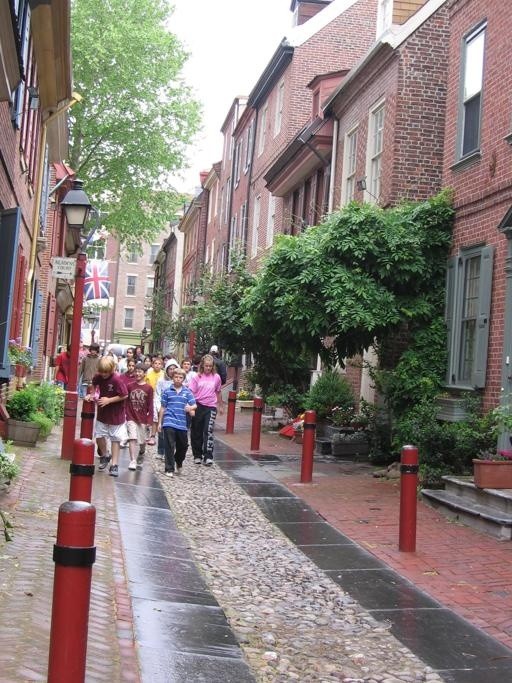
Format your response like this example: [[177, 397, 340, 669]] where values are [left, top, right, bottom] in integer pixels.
[[79, 343, 227, 477], [52, 342, 71, 390]]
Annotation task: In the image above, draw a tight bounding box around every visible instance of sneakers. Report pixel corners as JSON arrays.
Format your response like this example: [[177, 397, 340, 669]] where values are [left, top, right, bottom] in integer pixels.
[[109, 464, 119, 476], [155, 454, 182, 477], [145, 435, 156, 445], [128, 451, 145, 470], [194, 457, 213, 466], [99, 453, 112, 469]]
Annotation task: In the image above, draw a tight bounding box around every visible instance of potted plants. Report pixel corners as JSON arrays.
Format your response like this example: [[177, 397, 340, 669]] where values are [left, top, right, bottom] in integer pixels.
[[0, 453, 16, 495], [352, 415, 369, 432], [4, 393, 42, 448]]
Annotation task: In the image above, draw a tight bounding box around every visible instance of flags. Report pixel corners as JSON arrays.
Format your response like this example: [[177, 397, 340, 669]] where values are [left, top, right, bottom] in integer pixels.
[[83, 259, 110, 301]]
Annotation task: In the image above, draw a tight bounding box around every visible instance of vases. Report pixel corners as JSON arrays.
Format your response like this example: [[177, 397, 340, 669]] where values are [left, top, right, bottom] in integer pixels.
[[15, 365, 25, 377], [471, 458, 512, 489]]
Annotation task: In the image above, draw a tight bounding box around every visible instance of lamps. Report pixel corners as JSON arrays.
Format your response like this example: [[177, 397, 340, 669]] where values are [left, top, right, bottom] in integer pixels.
[[60, 170, 92, 229]]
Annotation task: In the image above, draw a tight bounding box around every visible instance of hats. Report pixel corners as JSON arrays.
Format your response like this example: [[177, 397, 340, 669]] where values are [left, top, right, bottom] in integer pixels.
[[210, 345, 219, 354]]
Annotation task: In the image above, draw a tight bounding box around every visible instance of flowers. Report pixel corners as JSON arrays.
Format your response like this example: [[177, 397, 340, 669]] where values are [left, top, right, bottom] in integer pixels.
[[476, 445, 512, 461], [7, 336, 34, 367]]
